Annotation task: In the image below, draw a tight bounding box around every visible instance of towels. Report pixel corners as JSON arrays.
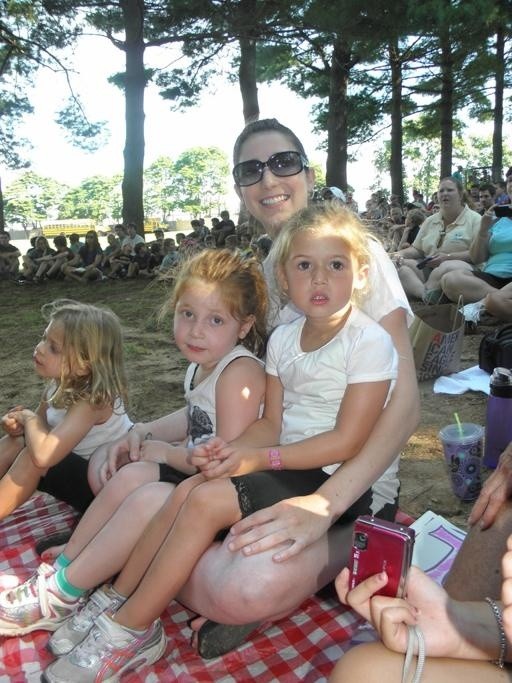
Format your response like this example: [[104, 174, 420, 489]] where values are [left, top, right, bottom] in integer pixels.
[[434, 361, 495, 401]]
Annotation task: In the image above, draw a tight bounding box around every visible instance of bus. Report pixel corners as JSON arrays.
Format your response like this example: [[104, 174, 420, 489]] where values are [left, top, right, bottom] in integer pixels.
[[40, 220, 110, 237]]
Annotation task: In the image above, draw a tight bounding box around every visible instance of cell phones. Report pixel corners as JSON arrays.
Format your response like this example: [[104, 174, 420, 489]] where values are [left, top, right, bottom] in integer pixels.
[[416, 256, 434, 270], [494, 205, 511, 217]]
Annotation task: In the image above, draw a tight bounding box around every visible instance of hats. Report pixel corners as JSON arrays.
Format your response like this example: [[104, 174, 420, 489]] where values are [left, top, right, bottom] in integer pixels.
[[320, 186, 346, 203], [406, 201, 424, 210]]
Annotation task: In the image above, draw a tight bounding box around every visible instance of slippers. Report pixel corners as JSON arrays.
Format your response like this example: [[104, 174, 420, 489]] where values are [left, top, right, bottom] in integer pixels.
[[36, 530, 72, 556], [199, 619, 261, 660]]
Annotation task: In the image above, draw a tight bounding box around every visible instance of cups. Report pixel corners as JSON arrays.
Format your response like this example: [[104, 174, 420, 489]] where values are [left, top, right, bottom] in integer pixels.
[[438, 423, 485, 503]]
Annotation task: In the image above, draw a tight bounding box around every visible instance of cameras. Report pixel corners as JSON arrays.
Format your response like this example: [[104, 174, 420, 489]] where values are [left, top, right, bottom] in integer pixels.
[[348, 514, 415, 597]]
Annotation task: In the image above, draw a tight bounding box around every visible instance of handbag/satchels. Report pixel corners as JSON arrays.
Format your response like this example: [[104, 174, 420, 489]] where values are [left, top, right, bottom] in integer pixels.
[[406, 278, 467, 385]]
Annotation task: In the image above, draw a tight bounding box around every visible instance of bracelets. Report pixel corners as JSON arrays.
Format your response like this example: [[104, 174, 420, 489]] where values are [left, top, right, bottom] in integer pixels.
[[267, 446, 282, 472], [480, 595, 505, 671]]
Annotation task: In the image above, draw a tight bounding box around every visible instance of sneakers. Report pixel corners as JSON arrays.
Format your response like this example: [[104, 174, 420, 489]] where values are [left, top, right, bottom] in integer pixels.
[[41, 606, 169, 683], [421, 287, 448, 306], [46, 582, 129, 656], [0, 563, 95, 636]]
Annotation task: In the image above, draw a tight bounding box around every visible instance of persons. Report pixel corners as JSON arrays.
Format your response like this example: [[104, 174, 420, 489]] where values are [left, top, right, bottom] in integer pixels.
[[321, 161, 512, 328], [440, 441, 512, 602], [330, 534, 512, 682], [32, 117, 425, 661], [40, 202, 398, 683], [0, 297, 137, 528], [0, 248, 270, 640], [0, 208, 273, 286]]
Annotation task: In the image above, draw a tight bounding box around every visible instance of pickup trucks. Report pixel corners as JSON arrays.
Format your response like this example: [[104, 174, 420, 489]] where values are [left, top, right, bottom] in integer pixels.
[[143, 219, 173, 232]]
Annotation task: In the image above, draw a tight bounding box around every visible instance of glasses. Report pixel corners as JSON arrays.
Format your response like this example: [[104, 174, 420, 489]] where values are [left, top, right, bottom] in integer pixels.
[[436, 230, 445, 248], [85, 236, 96, 239], [233, 151, 310, 188]]
